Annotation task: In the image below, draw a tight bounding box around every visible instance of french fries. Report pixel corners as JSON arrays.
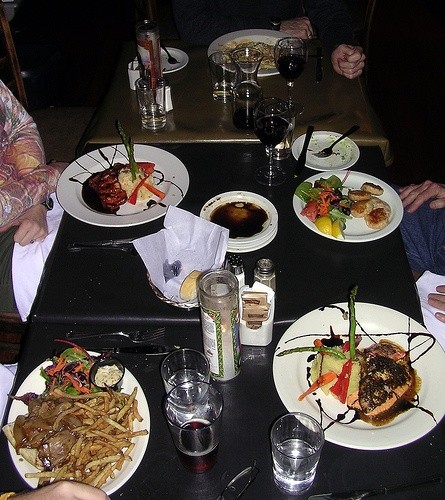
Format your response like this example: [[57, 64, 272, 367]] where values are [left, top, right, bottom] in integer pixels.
[[25, 388, 148, 490]]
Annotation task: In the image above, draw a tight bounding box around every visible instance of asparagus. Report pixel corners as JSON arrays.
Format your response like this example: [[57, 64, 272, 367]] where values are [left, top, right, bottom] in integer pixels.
[[276, 347, 345, 359], [116, 118, 140, 181], [349, 285, 358, 361]]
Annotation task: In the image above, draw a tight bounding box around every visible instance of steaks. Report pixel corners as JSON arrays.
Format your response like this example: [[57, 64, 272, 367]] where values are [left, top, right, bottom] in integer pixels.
[[89, 165, 129, 211]]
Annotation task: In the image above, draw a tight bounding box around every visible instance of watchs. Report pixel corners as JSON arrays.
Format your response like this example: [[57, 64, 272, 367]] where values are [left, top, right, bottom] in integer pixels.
[[270, 16, 281, 31], [42, 197, 53, 211]]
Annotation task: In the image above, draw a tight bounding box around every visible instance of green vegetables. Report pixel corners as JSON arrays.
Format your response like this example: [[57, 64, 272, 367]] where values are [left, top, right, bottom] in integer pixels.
[[294, 176, 353, 229], [40, 346, 102, 396]]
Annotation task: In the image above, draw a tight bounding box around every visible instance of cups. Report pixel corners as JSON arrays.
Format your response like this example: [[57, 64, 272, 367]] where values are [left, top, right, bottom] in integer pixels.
[[161, 350, 213, 422], [164, 379, 224, 474], [209, 50, 236, 104], [135, 74, 168, 130], [264, 97, 295, 160], [135, 18, 161, 80], [269, 411, 326, 495]]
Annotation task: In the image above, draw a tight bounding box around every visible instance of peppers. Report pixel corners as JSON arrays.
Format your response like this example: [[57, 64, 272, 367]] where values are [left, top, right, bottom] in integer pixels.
[[129, 176, 149, 204], [330, 335, 362, 403], [126, 161, 155, 173]]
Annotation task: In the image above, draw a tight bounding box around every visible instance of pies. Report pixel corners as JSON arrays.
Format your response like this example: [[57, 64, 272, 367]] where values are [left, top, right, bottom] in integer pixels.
[[2, 413, 44, 472]]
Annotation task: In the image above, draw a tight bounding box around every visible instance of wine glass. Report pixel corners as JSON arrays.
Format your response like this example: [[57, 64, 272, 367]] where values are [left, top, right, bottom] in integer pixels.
[[274, 36, 303, 117]]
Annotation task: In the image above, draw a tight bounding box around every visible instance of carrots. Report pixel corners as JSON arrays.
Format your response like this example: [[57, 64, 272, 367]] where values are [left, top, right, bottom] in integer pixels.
[[315, 339, 327, 354], [144, 183, 165, 204], [298, 372, 336, 400]]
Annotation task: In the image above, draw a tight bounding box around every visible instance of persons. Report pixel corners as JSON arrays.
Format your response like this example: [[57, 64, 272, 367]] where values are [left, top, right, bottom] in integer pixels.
[[0, 79, 70, 312], [399, 180, 445, 323], [173, 0, 366, 79]]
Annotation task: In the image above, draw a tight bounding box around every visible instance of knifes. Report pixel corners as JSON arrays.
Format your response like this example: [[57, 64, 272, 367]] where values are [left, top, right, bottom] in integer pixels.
[[294, 126, 314, 179], [314, 47, 324, 83]]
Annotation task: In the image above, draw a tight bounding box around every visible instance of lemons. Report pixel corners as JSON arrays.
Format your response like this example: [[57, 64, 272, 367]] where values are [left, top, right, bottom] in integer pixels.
[[315, 216, 346, 240]]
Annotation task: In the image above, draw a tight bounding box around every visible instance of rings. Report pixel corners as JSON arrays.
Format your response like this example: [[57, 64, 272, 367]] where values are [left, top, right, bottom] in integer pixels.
[[30, 240, 34, 243]]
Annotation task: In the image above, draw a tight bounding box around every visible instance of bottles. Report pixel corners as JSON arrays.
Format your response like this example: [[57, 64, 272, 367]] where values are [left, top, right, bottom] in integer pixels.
[[252, 99, 289, 186], [253, 258, 278, 295], [199, 270, 242, 381], [231, 47, 263, 131], [154, 79, 174, 113], [221, 255, 245, 288]]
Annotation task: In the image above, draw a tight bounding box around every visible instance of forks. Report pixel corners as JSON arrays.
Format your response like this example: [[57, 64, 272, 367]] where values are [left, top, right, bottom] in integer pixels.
[[313, 123, 362, 157]]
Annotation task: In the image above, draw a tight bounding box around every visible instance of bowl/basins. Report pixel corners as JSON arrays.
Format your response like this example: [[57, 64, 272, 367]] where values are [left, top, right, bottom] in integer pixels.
[[89, 358, 125, 392]]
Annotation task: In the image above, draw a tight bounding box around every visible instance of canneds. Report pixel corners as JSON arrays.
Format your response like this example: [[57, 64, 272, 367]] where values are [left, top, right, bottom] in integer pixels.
[[134, 20, 163, 91], [197, 268, 242, 381]]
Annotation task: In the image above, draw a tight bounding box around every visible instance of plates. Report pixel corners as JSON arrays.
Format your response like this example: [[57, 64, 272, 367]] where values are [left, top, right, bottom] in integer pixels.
[[207, 28, 296, 76], [200, 190, 280, 253], [156, 46, 188, 74], [273, 302, 445, 450], [6, 350, 150, 498], [292, 130, 361, 171], [292, 170, 404, 243], [56, 145, 190, 228]]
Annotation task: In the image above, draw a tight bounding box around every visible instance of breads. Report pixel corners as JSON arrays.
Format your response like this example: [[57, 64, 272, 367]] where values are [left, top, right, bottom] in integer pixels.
[[179, 270, 203, 300]]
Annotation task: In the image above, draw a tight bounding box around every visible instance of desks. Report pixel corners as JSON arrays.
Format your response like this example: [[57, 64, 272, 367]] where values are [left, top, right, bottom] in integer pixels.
[[0, 38, 445, 500]]
